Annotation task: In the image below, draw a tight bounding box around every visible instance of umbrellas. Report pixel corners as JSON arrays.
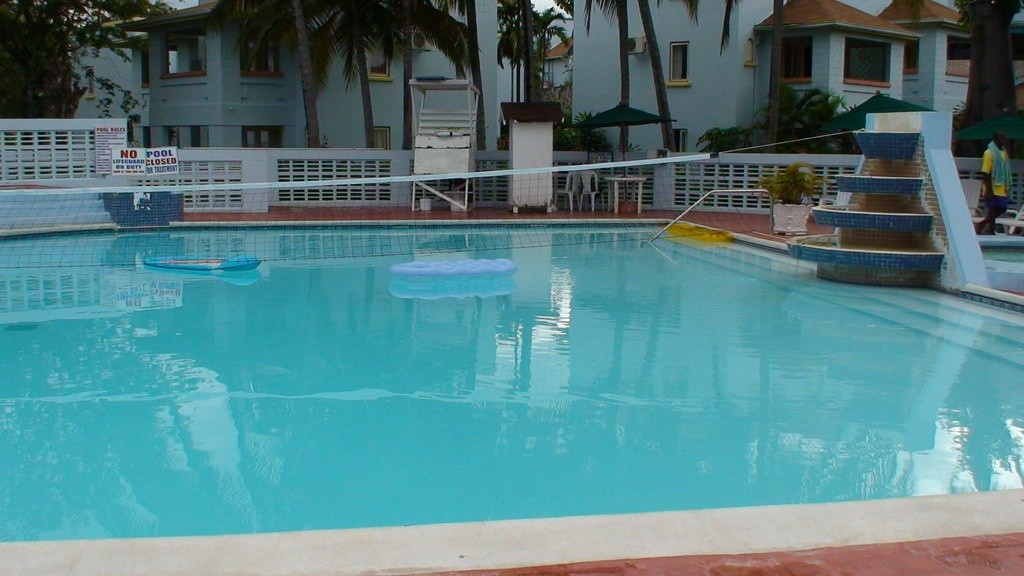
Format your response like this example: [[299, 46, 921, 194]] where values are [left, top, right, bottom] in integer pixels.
[[568, 101, 678, 212], [817, 89, 936, 132], [956, 109, 1024, 139]]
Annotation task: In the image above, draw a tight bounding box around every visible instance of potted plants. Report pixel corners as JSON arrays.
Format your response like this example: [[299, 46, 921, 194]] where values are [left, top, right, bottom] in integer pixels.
[[753, 161, 831, 237]]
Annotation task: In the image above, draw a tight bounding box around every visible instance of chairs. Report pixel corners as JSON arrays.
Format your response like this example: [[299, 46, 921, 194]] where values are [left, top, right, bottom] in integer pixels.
[[553, 171, 581, 212], [970, 203, 1024, 235], [578, 170, 604, 212]]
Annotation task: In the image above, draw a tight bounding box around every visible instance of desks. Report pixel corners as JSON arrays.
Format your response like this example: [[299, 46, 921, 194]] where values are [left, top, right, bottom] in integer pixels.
[[603, 174, 647, 214]]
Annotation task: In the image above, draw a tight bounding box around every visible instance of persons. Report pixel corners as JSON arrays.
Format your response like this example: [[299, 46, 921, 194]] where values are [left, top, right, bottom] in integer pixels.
[[972, 131, 1011, 235]]
